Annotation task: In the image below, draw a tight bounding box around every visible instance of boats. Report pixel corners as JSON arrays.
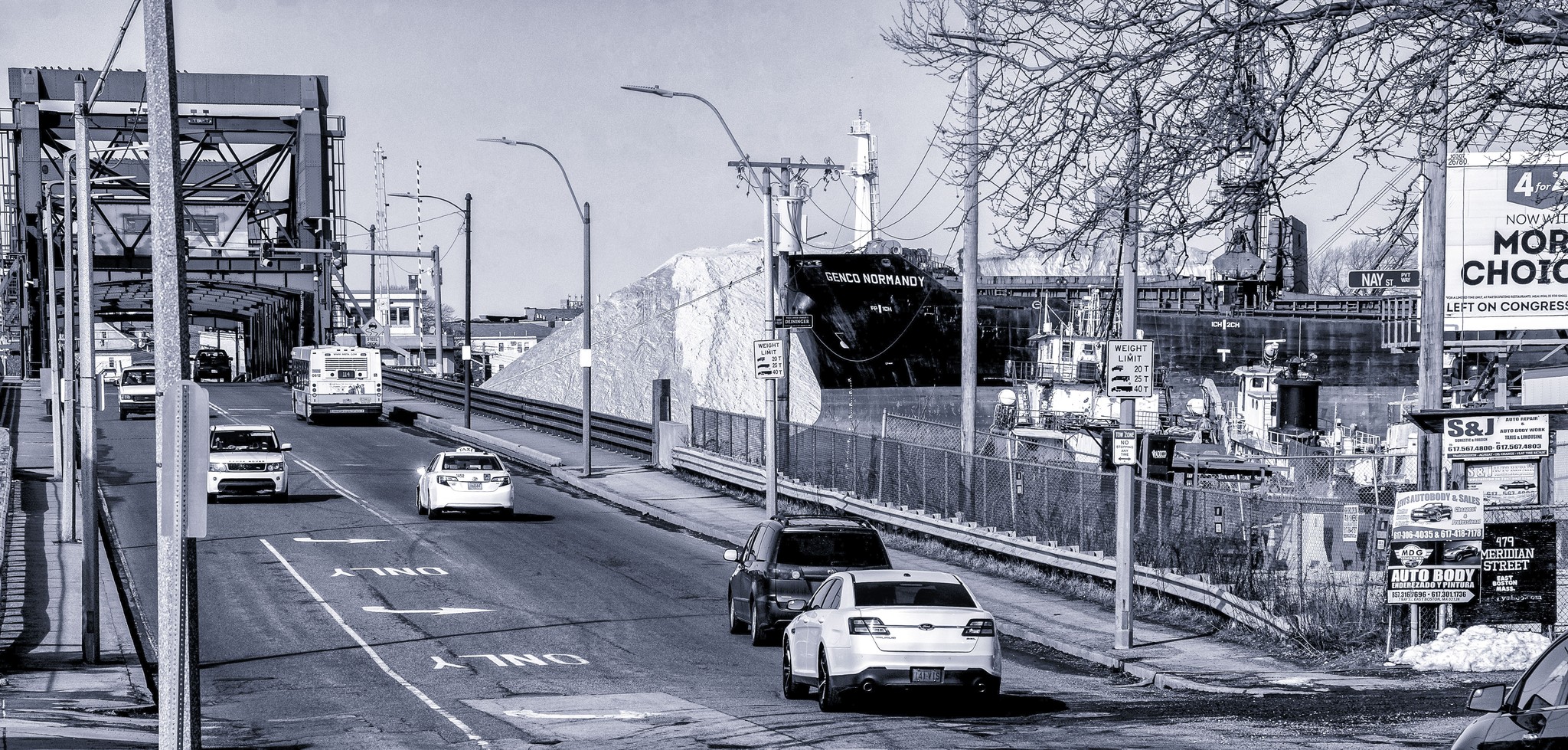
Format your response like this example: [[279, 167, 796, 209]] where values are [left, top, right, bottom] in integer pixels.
[[977, 280, 1417, 575]]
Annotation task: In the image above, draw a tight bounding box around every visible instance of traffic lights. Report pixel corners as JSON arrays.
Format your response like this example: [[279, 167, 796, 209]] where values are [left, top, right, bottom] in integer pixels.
[[347, 318, 356, 334], [331, 241, 342, 267], [260, 240, 273, 268]]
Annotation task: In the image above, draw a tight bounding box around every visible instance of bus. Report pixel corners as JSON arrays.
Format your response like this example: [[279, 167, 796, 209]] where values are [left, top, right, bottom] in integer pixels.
[[284, 338, 383, 424]]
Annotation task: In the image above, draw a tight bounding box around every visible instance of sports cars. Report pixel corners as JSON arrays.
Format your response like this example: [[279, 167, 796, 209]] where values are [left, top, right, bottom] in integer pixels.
[[1441, 545, 1478, 561], [1410, 502, 1453, 522]]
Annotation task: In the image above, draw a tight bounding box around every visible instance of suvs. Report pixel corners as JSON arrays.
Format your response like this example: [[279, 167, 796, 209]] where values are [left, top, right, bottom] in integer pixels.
[[722, 510, 897, 648], [207, 423, 292, 503], [113, 365, 156, 421], [193, 348, 234, 383]]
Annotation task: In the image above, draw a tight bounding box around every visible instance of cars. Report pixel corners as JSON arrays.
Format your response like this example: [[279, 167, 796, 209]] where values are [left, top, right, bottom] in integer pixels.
[[1449, 627, 1568, 750], [415, 445, 515, 521], [1499, 480, 1536, 490], [782, 568, 1002, 715]]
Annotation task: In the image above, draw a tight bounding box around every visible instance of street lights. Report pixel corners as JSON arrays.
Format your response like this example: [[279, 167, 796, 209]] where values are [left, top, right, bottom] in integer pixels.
[[58, 140, 194, 544], [309, 216, 376, 319], [474, 137, 593, 478], [388, 192, 472, 429], [44, 174, 138, 481], [621, 83, 777, 520]]
